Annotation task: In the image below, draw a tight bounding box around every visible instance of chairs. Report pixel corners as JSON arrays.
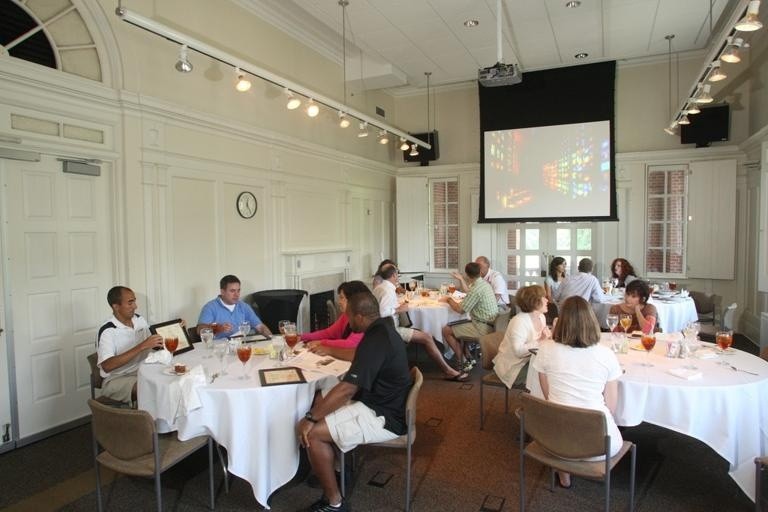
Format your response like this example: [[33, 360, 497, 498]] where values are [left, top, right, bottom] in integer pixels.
[[85, 283, 423, 512], [518, 392, 636, 512], [672, 286, 739, 338], [458, 289, 562, 431]]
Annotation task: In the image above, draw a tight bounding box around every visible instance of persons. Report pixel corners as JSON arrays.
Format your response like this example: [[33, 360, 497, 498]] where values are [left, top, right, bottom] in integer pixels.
[[298, 291, 413, 512], [197, 275, 272, 340], [296, 281, 372, 349], [97, 287, 187, 408]]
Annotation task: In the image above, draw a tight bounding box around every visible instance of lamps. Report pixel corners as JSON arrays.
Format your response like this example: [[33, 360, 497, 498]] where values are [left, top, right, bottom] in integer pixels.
[[663, 0, 763, 135], [166, 43, 421, 158]]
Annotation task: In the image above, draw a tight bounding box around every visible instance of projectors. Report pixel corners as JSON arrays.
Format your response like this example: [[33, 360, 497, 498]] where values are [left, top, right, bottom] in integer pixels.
[[478, 62, 524, 87]]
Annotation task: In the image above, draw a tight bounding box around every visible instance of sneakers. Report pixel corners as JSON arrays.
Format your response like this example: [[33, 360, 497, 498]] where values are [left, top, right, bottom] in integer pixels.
[[309, 463, 351, 488], [453, 358, 476, 372], [302, 496, 348, 512]]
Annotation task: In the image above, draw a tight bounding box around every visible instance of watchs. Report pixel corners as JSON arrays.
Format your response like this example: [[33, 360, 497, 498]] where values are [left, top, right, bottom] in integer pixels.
[[306, 412, 318, 423]]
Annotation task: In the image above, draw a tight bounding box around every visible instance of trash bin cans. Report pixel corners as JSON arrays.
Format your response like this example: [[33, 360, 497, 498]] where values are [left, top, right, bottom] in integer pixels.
[[755, 457, 768, 512]]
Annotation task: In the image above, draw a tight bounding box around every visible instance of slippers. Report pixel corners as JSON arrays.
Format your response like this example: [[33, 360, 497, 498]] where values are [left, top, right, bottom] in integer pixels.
[[558, 471, 572, 489], [444, 371, 471, 382]]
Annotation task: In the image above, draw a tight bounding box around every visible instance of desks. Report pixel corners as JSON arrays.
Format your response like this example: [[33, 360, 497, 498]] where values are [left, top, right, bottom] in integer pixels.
[[525, 331, 767, 504], [590, 293, 699, 334]]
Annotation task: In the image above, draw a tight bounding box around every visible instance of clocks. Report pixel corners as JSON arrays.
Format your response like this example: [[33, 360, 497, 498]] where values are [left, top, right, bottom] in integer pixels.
[[235, 190, 259, 219]]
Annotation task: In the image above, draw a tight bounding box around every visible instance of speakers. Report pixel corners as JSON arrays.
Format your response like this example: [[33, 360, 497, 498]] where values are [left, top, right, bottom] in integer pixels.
[[402, 131, 440, 163], [681, 101, 730, 142]]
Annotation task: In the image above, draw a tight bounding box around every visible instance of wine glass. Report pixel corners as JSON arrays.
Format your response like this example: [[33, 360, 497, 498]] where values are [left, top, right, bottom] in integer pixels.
[[399, 278, 457, 308], [159, 321, 326, 385], [599, 313, 734, 380], [599, 275, 678, 305]]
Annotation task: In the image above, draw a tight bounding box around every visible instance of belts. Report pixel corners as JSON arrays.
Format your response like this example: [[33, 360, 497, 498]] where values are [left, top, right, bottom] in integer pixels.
[[473, 318, 494, 328]]
